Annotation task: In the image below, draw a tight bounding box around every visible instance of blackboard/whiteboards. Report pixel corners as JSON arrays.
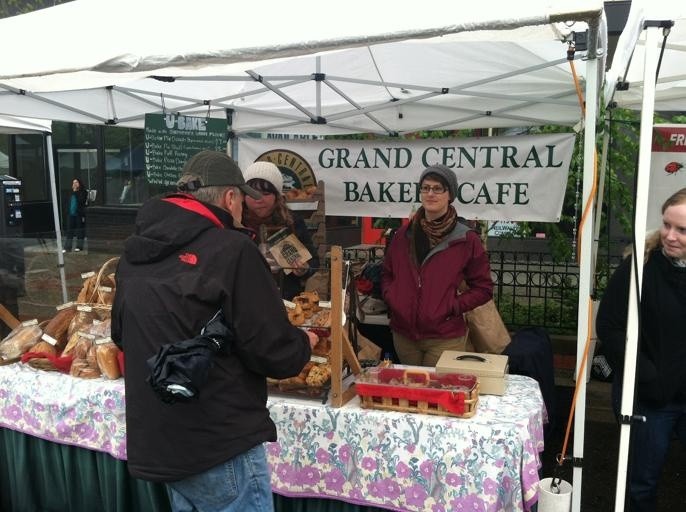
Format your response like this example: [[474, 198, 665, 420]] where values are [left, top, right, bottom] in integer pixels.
[[144, 114, 228, 186]]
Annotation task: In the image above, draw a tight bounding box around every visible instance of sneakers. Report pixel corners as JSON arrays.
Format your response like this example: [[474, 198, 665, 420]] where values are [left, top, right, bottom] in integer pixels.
[[62, 248, 80, 253]]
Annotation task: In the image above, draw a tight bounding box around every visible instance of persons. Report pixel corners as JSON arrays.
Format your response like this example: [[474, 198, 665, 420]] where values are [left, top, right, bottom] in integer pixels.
[[111, 150, 319, 512], [380, 164, 493, 366], [242, 161, 319, 302], [61, 178, 88, 253], [595, 188, 686, 512]]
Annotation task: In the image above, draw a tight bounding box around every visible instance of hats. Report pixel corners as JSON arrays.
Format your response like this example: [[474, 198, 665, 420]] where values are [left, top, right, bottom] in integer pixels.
[[418, 165, 458, 204], [178, 150, 283, 200]]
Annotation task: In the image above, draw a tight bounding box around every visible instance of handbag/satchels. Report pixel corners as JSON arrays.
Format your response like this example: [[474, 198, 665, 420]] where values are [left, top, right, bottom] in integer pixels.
[[462, 300, 511, 354]]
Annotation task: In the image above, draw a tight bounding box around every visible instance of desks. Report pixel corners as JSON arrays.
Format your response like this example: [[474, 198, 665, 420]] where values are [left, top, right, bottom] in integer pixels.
[[1, 355, 542, 512]]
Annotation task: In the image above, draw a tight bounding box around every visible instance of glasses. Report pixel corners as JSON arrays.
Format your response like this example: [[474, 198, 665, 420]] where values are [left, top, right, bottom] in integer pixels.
[[418, 184, 449, 194]]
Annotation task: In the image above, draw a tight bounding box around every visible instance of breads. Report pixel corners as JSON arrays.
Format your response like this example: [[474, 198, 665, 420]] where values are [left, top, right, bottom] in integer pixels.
[[0, 270, 120, 379], [266, 290, 333, 392]]
